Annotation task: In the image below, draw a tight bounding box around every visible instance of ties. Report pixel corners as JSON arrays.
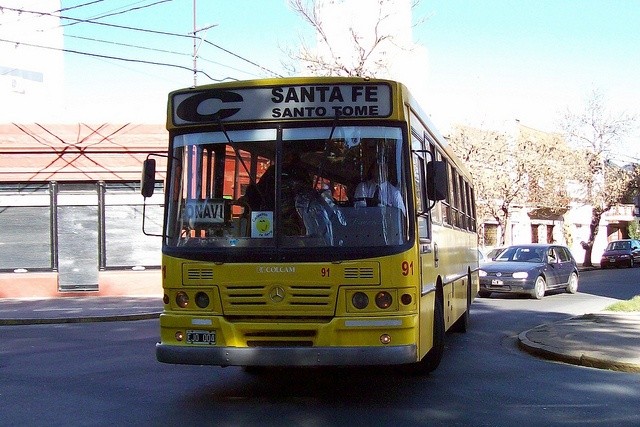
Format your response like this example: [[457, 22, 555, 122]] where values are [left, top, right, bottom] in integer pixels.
[[373, 185, 379, 199]]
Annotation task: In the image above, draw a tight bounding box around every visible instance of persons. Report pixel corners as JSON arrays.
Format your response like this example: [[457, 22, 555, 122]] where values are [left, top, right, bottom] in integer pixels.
[[354, 155, 407, 217]]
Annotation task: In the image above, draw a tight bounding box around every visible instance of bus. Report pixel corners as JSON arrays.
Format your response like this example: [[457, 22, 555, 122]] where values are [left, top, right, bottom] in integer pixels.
[[142, 78, 480, 372]]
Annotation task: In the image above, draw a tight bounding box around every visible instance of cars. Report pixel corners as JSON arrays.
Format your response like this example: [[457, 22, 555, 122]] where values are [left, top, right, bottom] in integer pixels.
[[601, 239, 640, 268], [479, 244, 578, 299]]
